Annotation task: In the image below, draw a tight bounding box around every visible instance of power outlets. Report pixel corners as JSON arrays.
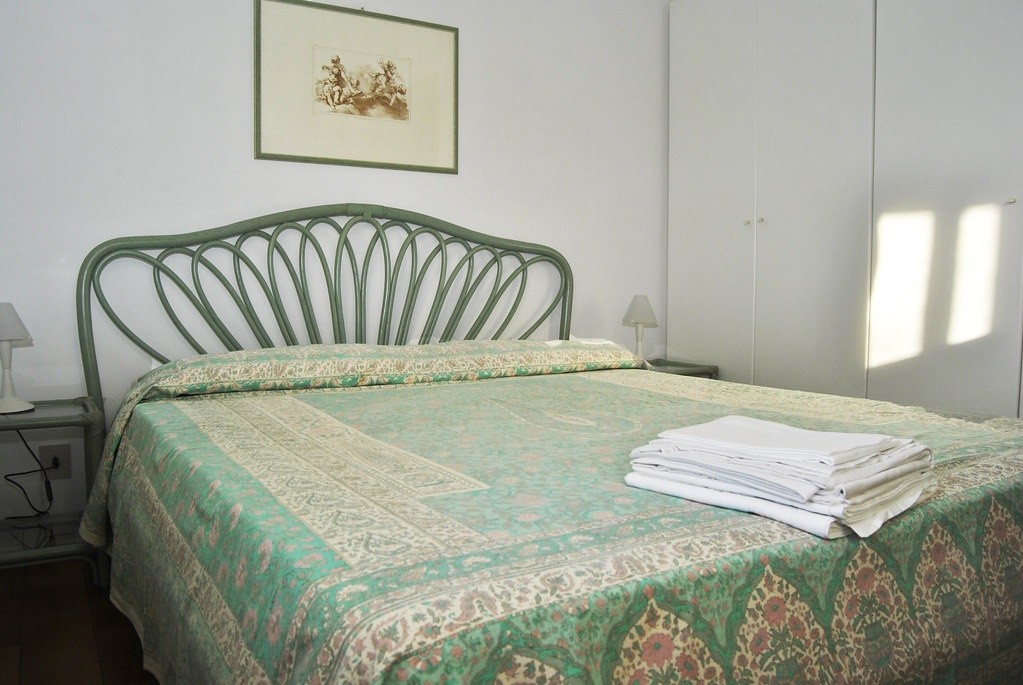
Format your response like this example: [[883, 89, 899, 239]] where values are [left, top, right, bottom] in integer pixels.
[[38, 444, 71, 481]]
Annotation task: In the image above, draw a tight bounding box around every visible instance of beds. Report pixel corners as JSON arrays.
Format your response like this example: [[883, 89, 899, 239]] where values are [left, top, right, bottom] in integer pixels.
[[74, 203, 1023, 685]]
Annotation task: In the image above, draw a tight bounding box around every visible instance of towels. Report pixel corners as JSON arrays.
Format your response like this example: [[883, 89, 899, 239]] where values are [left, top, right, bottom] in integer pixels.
[[625, 415, 937, 540]]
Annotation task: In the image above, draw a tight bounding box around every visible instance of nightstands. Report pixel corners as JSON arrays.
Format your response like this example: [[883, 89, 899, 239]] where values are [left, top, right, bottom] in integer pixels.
[[647, 358, 719, 379], [0, 394, 107, 590]]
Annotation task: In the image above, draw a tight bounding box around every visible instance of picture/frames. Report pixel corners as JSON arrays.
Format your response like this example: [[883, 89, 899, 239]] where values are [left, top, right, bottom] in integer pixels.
[[253, 0, 459, 175]]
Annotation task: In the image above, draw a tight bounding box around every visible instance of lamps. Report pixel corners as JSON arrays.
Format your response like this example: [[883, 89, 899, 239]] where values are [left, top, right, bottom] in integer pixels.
[[622, 294, 658, 354], [0, 303, 35, 414]]
